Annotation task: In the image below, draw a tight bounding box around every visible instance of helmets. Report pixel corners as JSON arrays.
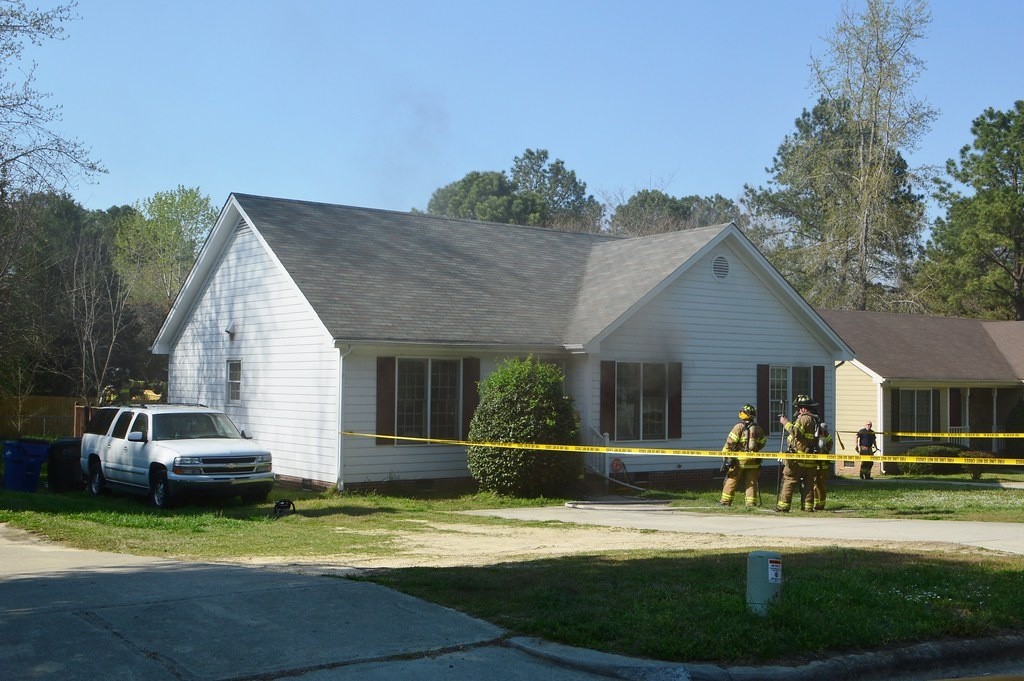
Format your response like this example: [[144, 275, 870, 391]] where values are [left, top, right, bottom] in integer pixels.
[[792, 394, 813, 407], [739, 403, 757, 419]]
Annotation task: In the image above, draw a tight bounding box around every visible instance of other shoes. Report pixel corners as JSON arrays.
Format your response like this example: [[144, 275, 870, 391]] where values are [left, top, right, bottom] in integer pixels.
[[716, 502, 730, 508], [813, 506, 824, 510], [774, 506, 789, 513], [865, 476, 874, 480], [859, 473, 864, 479], [801, 507, 814, 512]]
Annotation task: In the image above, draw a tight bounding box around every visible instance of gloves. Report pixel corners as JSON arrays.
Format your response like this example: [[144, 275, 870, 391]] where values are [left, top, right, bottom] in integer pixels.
[[720, 462, 729, 472]]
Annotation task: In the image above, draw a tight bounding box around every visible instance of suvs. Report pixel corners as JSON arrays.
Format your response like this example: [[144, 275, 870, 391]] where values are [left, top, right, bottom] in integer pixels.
[[79, 405, 276, 511]]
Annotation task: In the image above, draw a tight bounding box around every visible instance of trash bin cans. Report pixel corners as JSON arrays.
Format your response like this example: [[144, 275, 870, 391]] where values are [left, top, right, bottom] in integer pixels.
[[2, 439, 48, 494], [48, 438, 87, 493]]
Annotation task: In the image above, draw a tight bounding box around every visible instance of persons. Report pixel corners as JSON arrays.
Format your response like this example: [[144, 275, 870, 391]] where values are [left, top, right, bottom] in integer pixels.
[[773, 394, 833, 513], [715, 404, 766, 507], [855, 421, 879, 480]]
